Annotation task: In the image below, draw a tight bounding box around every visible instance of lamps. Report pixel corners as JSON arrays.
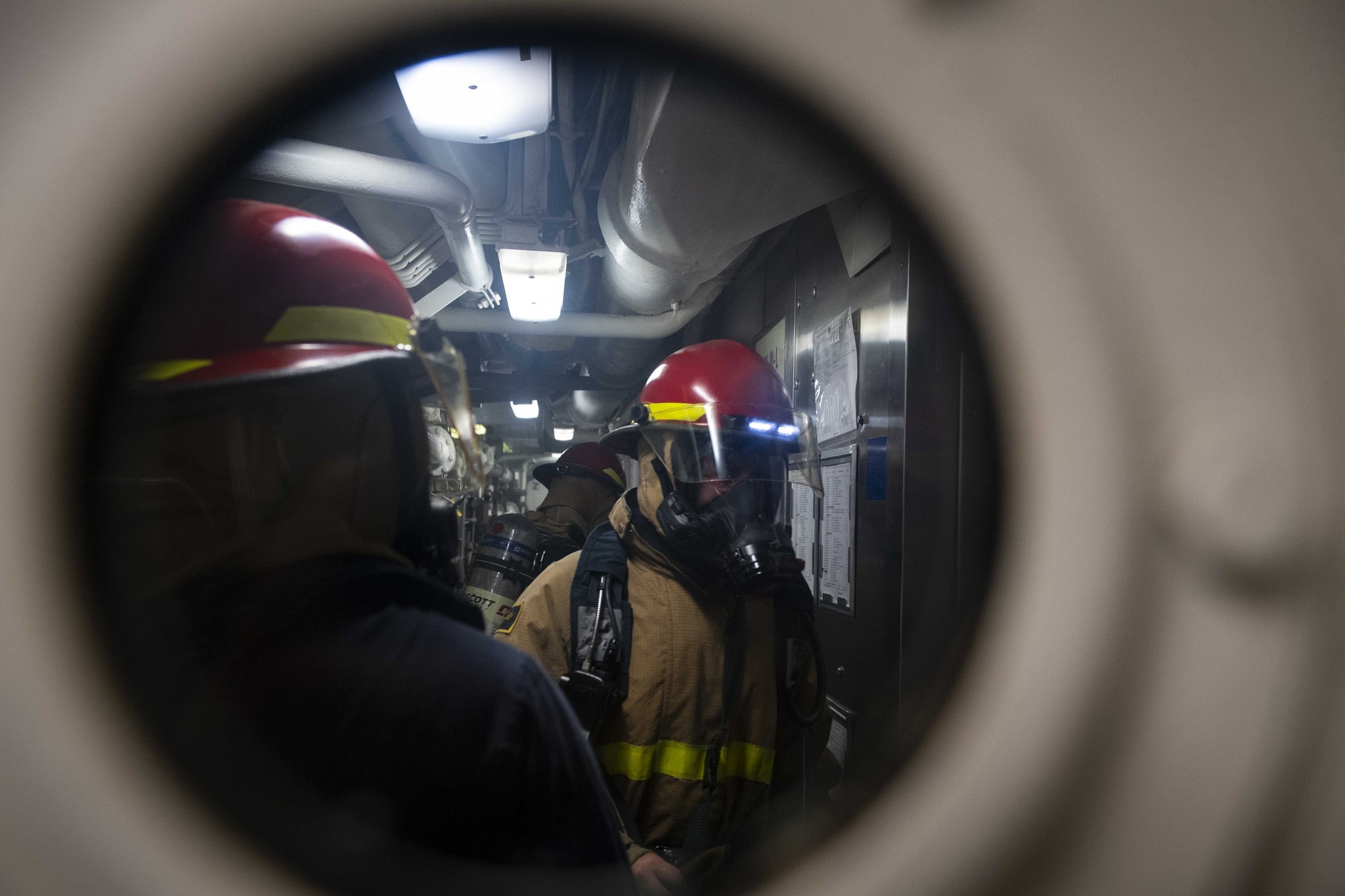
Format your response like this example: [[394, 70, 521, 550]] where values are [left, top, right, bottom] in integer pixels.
[[552, 427, 575, 442], [496, 245, 569, 326], [392, 44, 555, 145], [508, 398, 541, 420]]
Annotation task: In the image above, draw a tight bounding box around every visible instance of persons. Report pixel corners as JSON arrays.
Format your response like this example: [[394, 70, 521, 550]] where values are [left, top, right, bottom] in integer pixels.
[[111, 196, 650, 896], [461, 437, 631, 629], [502, 334, 842, 895]]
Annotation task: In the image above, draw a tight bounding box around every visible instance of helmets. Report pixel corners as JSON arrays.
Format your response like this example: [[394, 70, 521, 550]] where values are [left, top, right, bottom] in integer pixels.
[[107, 202, 489, 498], [532, 442, 627, 495], [599, 338, 827, 499]]
